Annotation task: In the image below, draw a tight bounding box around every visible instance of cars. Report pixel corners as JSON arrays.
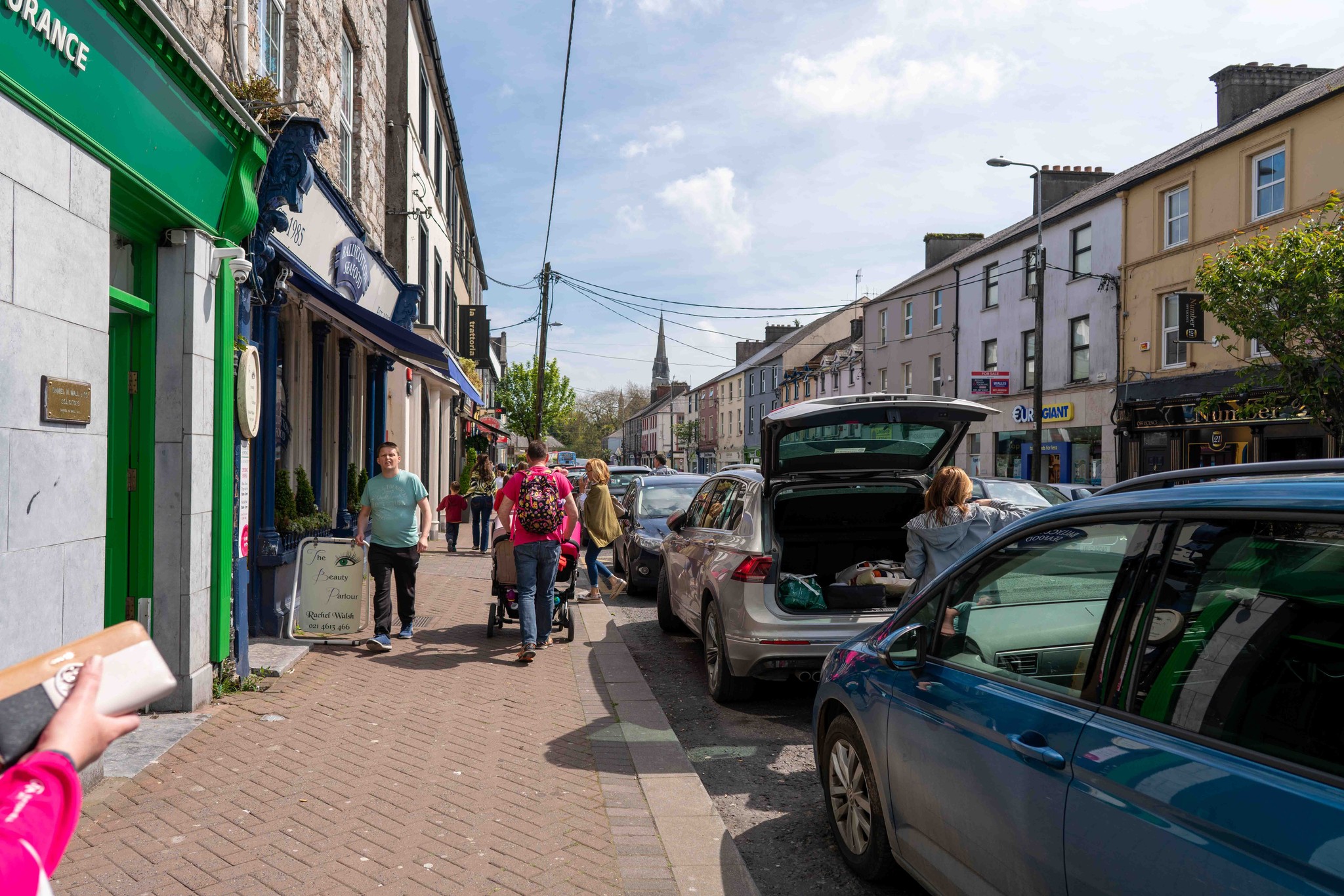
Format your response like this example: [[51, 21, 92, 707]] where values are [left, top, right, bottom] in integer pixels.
[[809, 475, 1344, 896], [547, 461, 698, 546], [613, 474, 713, 597], [967, 456, 1344, 579]]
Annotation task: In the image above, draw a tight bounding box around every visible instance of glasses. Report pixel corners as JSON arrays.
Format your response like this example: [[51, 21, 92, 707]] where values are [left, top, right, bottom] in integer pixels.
[[583, 471, 588, 479], [477, 454, 481, 460], [512, 470, 515, 471]]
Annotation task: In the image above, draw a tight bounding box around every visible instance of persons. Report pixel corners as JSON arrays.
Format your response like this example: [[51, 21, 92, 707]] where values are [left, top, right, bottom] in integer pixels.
[[436, 453, 528, 554], [703, 502, 723, 528], [0, 653, 141, 896], [677, 468, 682, 472], [647, 454, 679, 476], [355, 441, 432, 650], [493, 440, 579, 660], [1052, 464, 1060, 479], [577, 458, 627, 604], [897, 466, 1028, 611], [940, 593, 997, 638], [678, 539, 715, 592]]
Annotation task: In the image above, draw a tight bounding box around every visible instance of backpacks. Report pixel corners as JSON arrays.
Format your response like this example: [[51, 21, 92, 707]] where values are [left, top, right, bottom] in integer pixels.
[[516, 468, 565, 534]]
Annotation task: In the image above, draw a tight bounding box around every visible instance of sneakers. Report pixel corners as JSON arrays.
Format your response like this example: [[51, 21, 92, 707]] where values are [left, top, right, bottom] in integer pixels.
[[577, 593, 602, 603], [609, 578, 627, 600], [480, 550, 486, 554], [516, 643, 536, 663], [366, 634, 392, 650], [398, 622, 414, 638], [535, 638, 553, 649], [472, 545, 479, 550]]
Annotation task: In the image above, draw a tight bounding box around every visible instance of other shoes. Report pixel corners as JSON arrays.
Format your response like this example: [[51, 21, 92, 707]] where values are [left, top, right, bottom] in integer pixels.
[[447, 539, 452, 551], [453, 546, 456, 552]]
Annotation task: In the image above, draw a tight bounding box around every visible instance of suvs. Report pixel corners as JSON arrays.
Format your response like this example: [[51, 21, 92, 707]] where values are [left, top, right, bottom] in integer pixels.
[[655, 394, 1004, 705]]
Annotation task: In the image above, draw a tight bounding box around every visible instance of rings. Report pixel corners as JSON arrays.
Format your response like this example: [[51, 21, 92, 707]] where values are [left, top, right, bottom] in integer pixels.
[[357, 542, 358, 544]]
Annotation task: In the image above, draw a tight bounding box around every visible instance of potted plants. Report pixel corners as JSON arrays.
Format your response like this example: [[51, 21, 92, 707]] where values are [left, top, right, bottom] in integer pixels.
[[458, 447, 478, 523]]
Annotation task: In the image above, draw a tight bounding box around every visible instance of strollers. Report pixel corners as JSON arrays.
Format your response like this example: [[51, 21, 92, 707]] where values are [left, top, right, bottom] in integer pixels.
[[487, 514, 582, 641]]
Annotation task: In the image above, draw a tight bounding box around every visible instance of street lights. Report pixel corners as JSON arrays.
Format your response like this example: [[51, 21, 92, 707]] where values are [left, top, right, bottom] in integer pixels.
[[535, 322, 565, 361], [612, 405, 625, 466], [655, 376, 674, 470], [985, 154, 1047, 483]]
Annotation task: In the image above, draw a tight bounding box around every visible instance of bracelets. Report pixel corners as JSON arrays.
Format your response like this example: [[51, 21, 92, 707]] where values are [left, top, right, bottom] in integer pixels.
[[506, 532, 511, 535], [580, 492, 583, 495]]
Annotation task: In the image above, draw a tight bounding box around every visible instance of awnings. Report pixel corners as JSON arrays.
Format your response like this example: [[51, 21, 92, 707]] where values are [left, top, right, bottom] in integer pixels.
[[274, 263, 485, 408]]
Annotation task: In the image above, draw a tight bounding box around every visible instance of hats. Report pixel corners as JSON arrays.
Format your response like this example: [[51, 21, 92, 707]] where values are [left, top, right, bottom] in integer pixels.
[[497, 463, 507, 471]]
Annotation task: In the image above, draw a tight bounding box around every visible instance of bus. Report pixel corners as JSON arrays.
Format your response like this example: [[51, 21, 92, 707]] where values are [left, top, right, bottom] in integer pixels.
[[546, 451, 577, 467]]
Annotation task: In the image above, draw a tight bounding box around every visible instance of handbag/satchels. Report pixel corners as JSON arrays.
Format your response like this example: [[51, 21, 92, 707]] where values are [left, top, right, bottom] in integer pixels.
[[848, 569, 916, 586], [835, 559, 904, 584], [0, 620, 179, 773], [610, 495, 625, 517], [779, 573, 806, 582], [777, 577, 827, 610]]
